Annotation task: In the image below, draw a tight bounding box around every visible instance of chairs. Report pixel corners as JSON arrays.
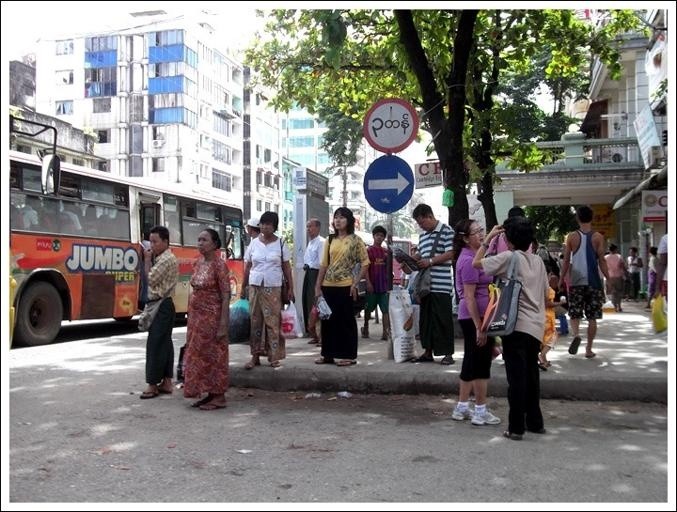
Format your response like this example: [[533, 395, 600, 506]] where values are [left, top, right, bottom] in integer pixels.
[[10, 203, 99, 235]]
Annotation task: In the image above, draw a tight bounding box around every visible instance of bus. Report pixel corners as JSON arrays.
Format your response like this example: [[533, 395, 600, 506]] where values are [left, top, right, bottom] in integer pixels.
[[384, 239, 414, 288], [9, 148, 249, 349]]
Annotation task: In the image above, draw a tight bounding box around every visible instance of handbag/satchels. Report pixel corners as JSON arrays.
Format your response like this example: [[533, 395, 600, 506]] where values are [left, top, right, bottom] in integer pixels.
[[408, 266, 432, 305], [137, 298, 163, 332], [279, 300, 304, 339], [649, 294, 668, 335], [227, 299, 250, 345], [480, 252, 524, 336]]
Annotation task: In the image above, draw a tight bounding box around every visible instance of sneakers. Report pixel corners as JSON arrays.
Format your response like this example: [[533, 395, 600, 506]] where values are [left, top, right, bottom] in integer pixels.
[[471, 410, 501, 426], [451, 406, 474, 421], [614, 293, 653, 313], [308, 326, 389, 347]]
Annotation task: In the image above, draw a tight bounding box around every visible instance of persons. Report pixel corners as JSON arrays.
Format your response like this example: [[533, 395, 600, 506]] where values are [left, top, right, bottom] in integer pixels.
[[139, 232, 153, 311], [135, 225, 179, 398], [245, 218, 262, 239], [448, 218, 503, 426], [183, 227, 231, 411], [239, 210, 295, 369], [10, 191, 131, 242], [302, 203, 667, 372], [472, 215, 550, 442]]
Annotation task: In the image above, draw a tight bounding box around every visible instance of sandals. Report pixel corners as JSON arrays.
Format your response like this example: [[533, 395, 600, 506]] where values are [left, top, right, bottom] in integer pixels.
[[245, 358, 281, 370], [526, 426, 547, 435], [503, 430, 522, 441]]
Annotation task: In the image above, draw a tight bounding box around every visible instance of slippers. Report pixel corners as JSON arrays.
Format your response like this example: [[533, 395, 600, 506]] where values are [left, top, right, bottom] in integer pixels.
[[200, 401, 228, 411], [314, 354, 356, 367], [140, 388, 160, 400], [410, 355, 436, 364], [440, 357, 456, 366], [537, 353, 552, 373], [157, 385, 173, 394], [567, 336, 597, 358], [191, 397, 213, 408]]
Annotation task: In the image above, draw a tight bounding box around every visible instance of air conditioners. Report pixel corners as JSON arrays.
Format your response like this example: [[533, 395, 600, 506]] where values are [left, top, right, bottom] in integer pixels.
[[644, 145, 660, 170], [153, 140, 161, 147]]
